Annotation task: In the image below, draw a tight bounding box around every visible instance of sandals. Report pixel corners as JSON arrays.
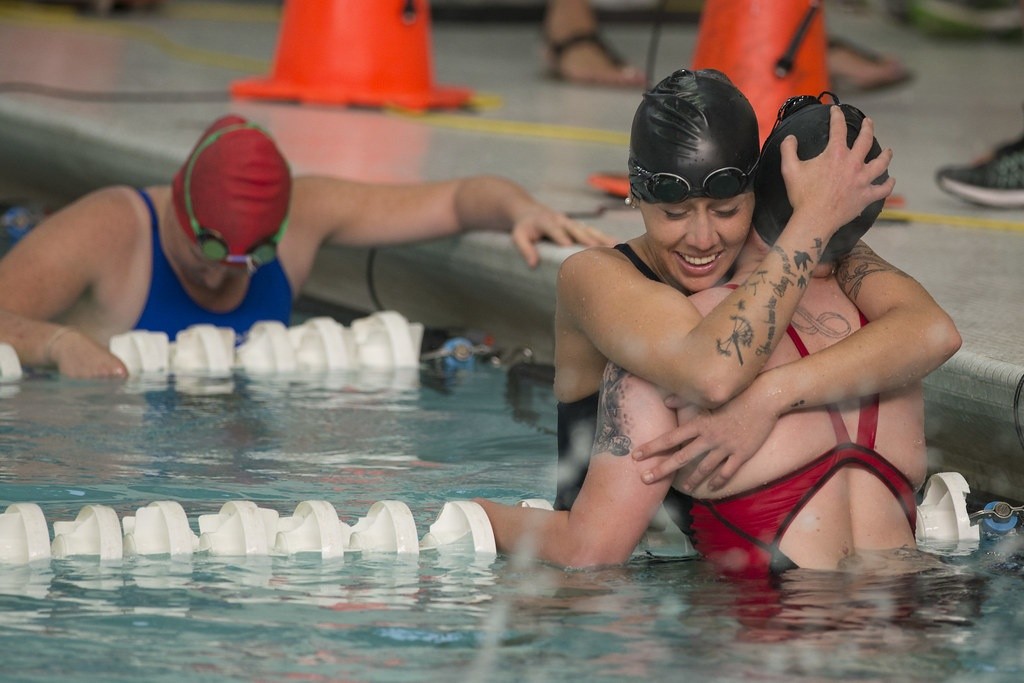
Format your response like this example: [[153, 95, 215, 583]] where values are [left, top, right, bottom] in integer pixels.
[[829, 36, 910, 95], [543, 28, 636, 87]]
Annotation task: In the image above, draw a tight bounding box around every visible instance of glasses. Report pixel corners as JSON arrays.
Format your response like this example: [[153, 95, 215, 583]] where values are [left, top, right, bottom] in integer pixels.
[[630, 163, 748, 203], [778, 90, 866, 121], [195, 228, 277, 266]]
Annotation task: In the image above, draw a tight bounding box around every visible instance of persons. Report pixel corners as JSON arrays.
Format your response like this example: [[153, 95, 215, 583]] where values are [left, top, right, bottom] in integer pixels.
[[472, 67, 963, 574], [536, 1, 655, 91], [0, 113, 619, 381]]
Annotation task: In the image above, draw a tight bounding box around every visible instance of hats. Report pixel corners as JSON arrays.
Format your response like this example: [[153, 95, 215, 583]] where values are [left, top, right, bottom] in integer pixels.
[[172, 116, 292, 266], [752, 103, 889, 262], [628, 69, 760, 203]]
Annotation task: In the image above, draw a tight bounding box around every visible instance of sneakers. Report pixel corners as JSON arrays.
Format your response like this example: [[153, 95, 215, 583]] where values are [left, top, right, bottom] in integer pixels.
[[938, 136, 1024, 209]]
